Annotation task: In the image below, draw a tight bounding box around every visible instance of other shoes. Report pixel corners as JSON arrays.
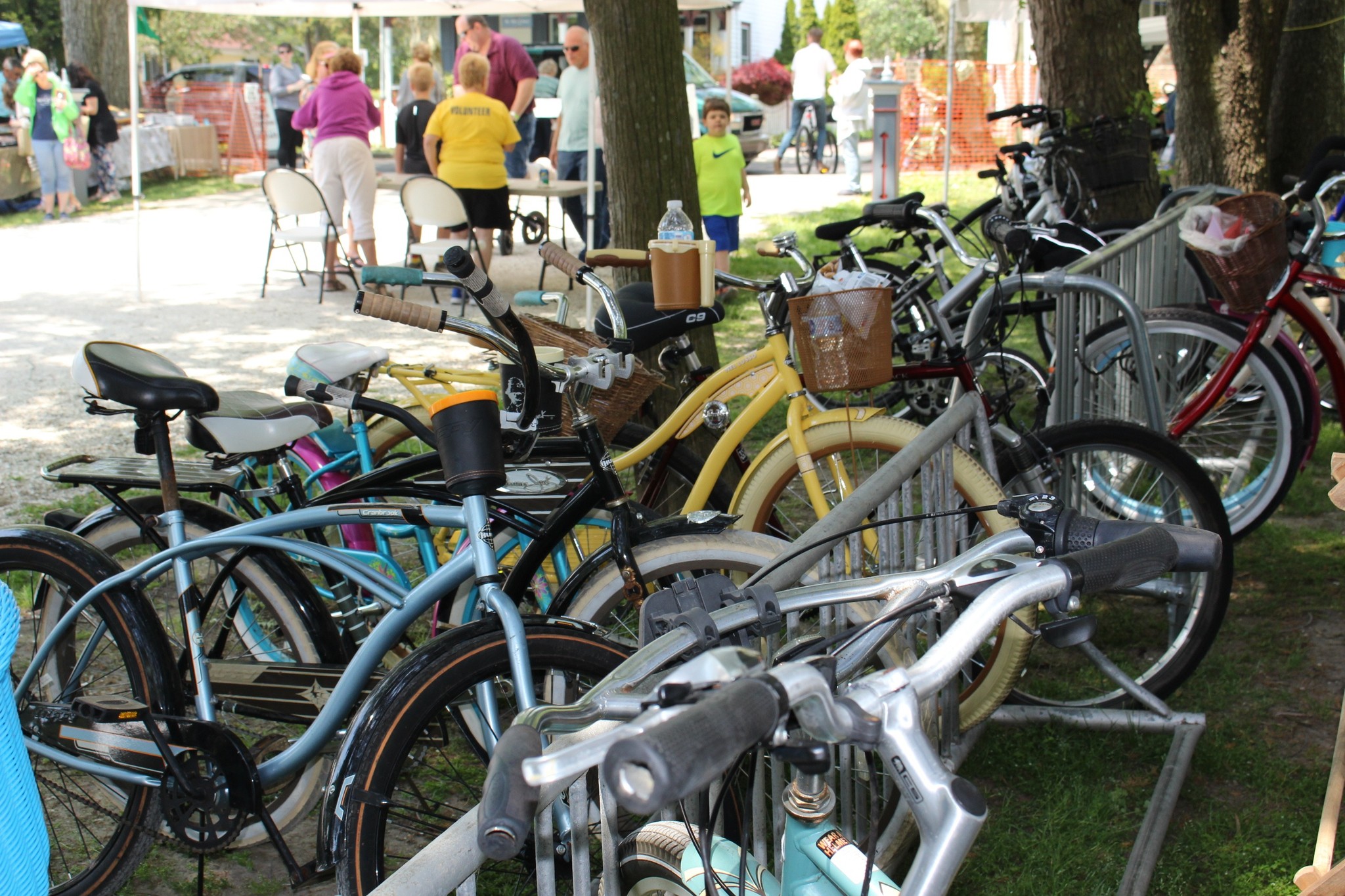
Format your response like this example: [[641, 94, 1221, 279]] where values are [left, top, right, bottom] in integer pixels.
[[100, 189, 121, 203], [59, 211, 70, 220], [44, 213, 56, 221], [773, 159, 782, 174]]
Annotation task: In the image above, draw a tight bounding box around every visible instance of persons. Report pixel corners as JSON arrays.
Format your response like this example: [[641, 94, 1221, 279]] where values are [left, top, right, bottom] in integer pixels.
[[771, 26, 874, 197], [266, 13, 752, 298], [1, 47, 123, 223]]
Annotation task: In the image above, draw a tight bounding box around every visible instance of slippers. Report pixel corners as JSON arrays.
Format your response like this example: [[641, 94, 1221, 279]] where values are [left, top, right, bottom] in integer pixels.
[[347, 256, 365, 268], [333, 264, 353, 275]]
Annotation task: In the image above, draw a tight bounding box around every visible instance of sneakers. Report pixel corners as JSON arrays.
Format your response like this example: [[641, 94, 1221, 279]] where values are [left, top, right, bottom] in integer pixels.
[[714, 286, 738, 302], [409, 263, 423, 271], [434, 262, 451, 273], [451, 287, 469, 304]]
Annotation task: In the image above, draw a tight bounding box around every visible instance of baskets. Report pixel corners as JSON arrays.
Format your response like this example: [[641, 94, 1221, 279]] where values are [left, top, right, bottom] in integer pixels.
[[1185, 192, 1290, 313], [519, 312, 665, 448], [787, 288, 896, 392]]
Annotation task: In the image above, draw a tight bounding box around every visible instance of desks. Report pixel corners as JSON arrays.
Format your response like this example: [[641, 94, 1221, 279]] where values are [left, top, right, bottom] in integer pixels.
[[231, 172, 602, 313], [0, 121, 39, 214], [89, 125, 225, 192]]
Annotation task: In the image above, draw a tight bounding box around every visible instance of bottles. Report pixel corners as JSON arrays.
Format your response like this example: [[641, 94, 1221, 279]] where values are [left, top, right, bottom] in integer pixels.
[[657, 200, 694, 241], [810, 287, 851, 391]]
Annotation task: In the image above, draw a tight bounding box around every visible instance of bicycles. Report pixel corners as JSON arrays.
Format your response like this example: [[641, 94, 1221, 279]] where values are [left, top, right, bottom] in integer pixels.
[[795, 103, 840, 173], [820, 101, 1345, 719], [0, 242, 966, 896]]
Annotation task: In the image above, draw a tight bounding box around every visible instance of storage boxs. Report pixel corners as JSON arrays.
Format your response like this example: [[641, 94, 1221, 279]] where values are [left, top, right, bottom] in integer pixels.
[[650, 238, 718, 312]]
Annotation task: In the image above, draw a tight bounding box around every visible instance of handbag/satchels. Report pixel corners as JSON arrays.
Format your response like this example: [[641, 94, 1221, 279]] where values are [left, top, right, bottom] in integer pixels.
[[64, 122, 91, 170]]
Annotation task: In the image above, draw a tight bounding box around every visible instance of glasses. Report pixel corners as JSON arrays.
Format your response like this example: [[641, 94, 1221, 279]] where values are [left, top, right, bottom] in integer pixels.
[[280, 50, 291, 54], [320, 61, 329, 68], [459, 27, 472, 38], [563, 45, 579, 53]]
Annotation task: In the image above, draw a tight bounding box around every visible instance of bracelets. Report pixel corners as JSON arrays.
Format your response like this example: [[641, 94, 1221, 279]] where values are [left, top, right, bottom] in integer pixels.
[[510, 110, 520, 122]]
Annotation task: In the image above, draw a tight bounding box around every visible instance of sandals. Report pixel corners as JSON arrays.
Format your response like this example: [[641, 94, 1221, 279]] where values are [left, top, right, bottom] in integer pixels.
[[323, 280, 345, 291], [366, 283, 394, 298]]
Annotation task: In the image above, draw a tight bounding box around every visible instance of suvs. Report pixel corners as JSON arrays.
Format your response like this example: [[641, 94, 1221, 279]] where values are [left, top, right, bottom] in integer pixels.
[[149, 62, 272, 98], [523, 43, 762, 165]]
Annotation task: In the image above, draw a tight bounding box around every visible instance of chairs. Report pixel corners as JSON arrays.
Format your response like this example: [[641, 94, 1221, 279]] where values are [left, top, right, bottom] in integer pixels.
[[261, 168, 363, 303], [399, 176, 494, 315]]
